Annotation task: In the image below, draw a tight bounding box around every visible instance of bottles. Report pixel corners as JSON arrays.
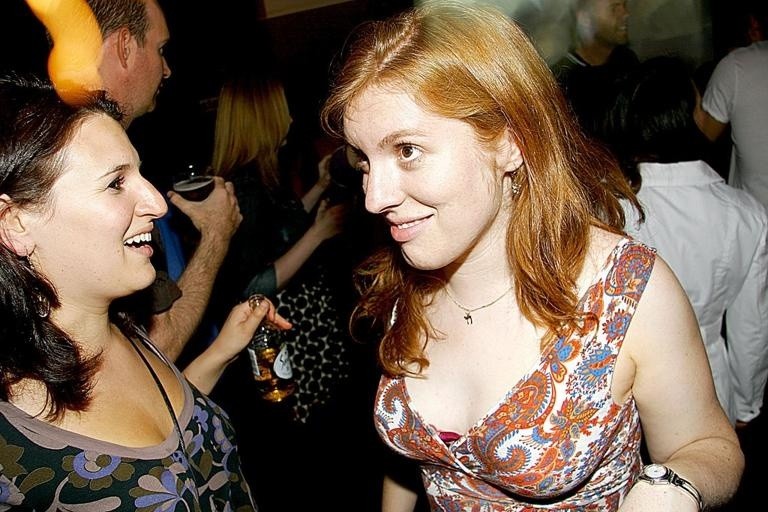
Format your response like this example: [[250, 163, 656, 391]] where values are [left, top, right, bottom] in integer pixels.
[[246, 293, 297, 403]]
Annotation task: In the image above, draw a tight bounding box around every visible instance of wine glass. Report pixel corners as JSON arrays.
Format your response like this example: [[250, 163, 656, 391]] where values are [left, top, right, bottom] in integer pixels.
[[169, 164, 219, 205]]
